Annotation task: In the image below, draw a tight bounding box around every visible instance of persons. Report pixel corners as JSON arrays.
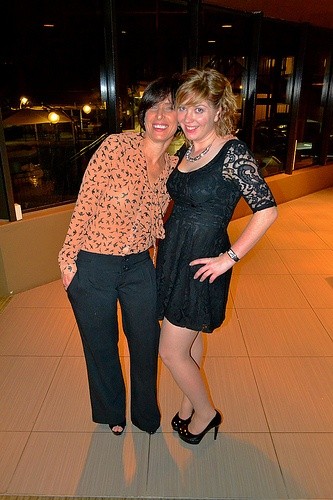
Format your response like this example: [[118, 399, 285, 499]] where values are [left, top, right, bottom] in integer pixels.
[[59, 78, 179, 438], [160, 66, 280, 445]]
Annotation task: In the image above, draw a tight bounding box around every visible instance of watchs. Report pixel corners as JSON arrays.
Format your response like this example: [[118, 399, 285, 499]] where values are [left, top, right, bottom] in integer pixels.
[[228, 248, 240, 263]]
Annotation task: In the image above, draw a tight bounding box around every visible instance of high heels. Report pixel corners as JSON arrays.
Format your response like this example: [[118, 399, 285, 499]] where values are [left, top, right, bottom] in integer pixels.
[[171, 408, 221, 445], [109, 423, 155, 436]]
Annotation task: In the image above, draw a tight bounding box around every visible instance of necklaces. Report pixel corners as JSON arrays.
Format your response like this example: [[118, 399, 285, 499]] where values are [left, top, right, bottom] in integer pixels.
[[186, 136, 219, 164]]
